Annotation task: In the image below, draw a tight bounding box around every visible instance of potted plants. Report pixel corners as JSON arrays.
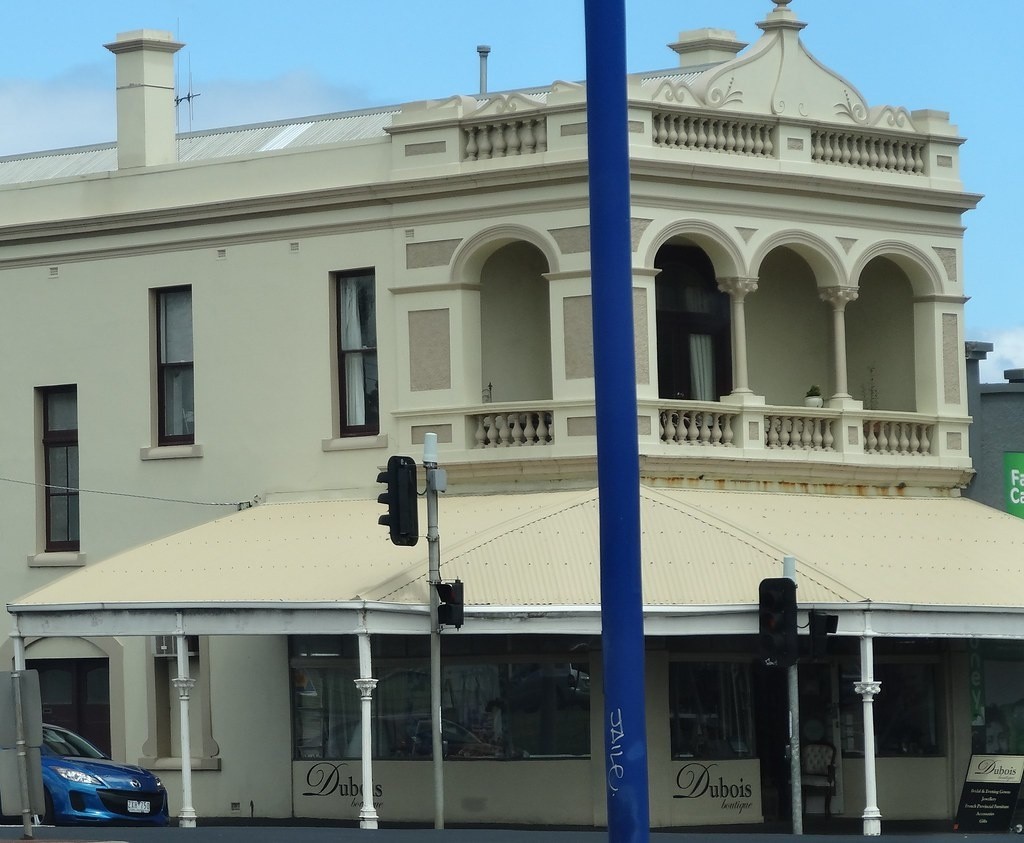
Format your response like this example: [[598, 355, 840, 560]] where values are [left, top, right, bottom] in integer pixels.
[[805, 385, 824, 407]]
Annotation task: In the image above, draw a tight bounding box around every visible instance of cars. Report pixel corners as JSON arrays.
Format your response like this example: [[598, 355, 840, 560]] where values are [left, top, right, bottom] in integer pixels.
[[0, 723, 170, 828], [508, 656, 591, 709], [296, 714, 531, 761]]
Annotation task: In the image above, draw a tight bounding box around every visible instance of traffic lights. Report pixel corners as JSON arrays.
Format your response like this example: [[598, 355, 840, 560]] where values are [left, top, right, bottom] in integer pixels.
[[808, 611, 839, 641], [758, 577, 798, 666], [436, 580, 464, 628], [376, 455, 418, 546]]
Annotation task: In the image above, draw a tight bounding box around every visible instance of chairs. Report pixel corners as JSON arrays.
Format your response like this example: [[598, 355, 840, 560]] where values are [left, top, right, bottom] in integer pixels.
[[802, 742, 837, 821]]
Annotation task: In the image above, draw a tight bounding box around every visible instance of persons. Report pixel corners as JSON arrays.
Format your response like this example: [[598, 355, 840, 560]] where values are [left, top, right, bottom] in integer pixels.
[[985, 705, 1010, 755]]
